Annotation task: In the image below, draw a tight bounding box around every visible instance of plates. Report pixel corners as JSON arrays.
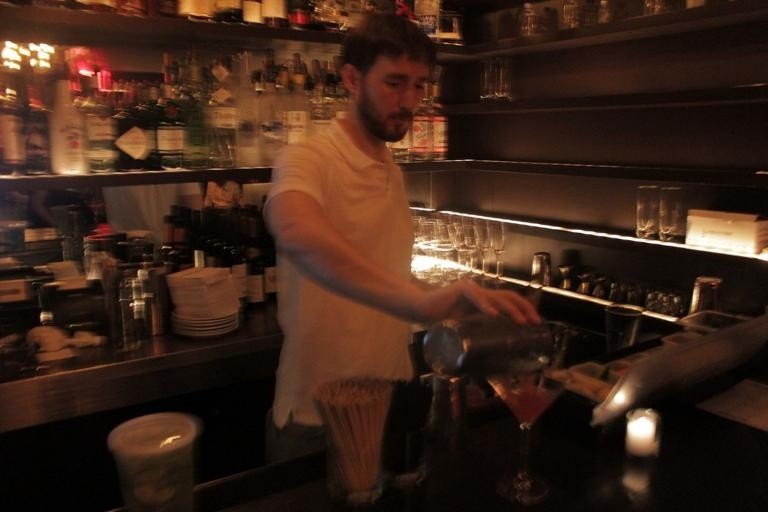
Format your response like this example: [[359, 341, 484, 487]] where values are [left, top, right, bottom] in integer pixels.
[[170, 309, 241, 339]]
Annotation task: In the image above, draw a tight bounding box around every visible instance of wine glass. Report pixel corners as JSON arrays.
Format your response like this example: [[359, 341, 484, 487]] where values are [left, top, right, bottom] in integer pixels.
[[408, 213, 515, 288], [485, 372, 566, 504]]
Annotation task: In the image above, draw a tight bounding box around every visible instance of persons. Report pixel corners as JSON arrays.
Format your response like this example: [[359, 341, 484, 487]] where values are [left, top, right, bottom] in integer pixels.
[[262, 9, 546, 461]]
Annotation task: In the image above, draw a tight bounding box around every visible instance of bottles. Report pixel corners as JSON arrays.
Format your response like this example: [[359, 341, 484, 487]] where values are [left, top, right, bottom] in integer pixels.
[[559, 0, 582, 29], [0, 44, 449, 176], [530, 251, 553, 288], [518, 2, 541, 37], [162, 205, 277, 311], [598, 0, 614, 24]]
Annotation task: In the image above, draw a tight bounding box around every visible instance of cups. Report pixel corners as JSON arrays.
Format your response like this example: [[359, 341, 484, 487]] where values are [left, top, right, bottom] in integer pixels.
[[684, 276, 725, 330], [422, 315, 553, 379], [603, 302, 645, 351], [636, 185, 686, 242], [479, 57, 513, 106], [646, 286, 685, 316], [107, 412, 198, 511]]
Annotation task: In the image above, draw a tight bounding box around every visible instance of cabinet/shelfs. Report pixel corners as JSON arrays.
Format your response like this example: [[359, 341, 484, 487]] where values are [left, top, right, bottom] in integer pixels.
[[0, 0, 768, 330]]
